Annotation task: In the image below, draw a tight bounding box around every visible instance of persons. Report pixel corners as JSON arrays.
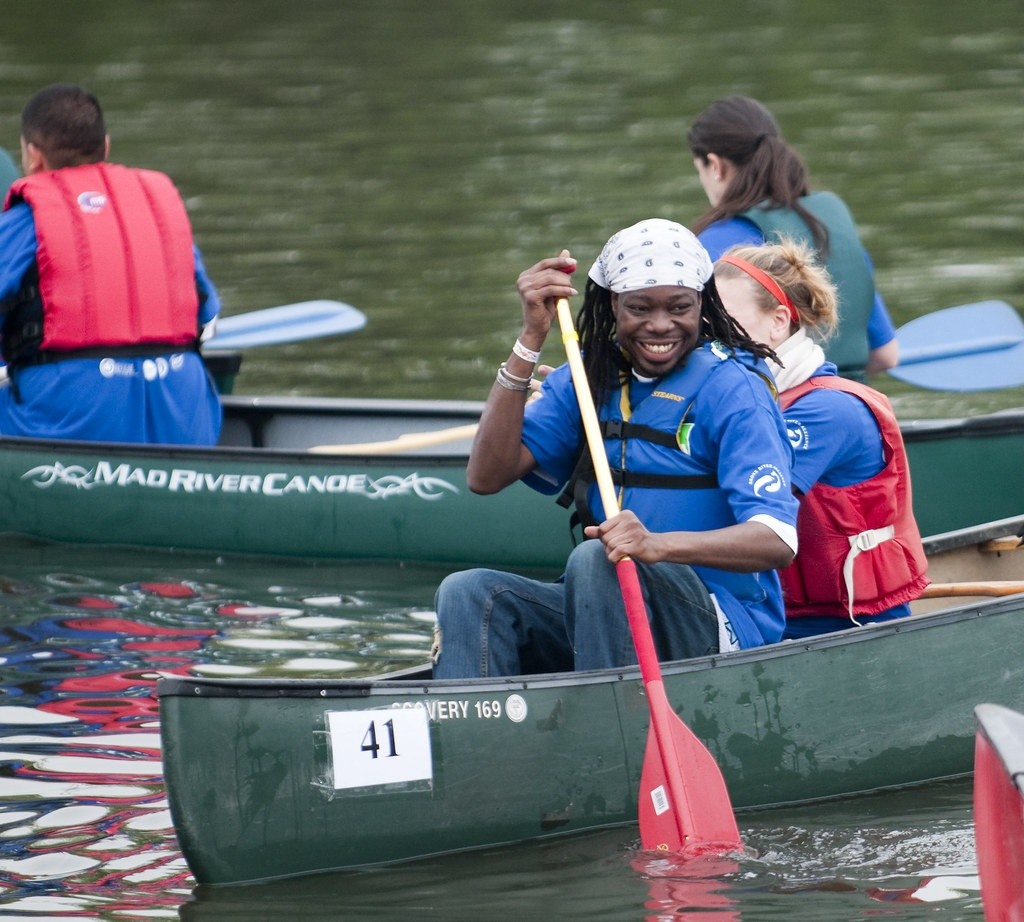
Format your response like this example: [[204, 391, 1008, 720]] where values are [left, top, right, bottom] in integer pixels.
[[0, 84, 222, 447], [431, 218, 801, 680], [712, 245, 932, 642], [687, 95, 900, 386]]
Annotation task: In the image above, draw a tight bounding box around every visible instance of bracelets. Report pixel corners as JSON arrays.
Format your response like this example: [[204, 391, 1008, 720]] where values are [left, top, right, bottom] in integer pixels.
[[512, 337, 541, 364], [496, 367, 534, 390]]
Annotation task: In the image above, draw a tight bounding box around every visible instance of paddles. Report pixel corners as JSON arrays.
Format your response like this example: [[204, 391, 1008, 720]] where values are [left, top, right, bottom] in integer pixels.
[[913, 580, 1024, 599], [201, 295, 369, 358], [311, 298, 1024, 455], [557, 295, 743, 855]]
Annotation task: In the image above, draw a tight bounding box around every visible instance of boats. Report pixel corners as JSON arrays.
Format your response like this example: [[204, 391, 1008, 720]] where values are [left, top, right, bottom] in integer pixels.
[[156, 515, 1024, 921], [0, 398, 1024, 565]]
